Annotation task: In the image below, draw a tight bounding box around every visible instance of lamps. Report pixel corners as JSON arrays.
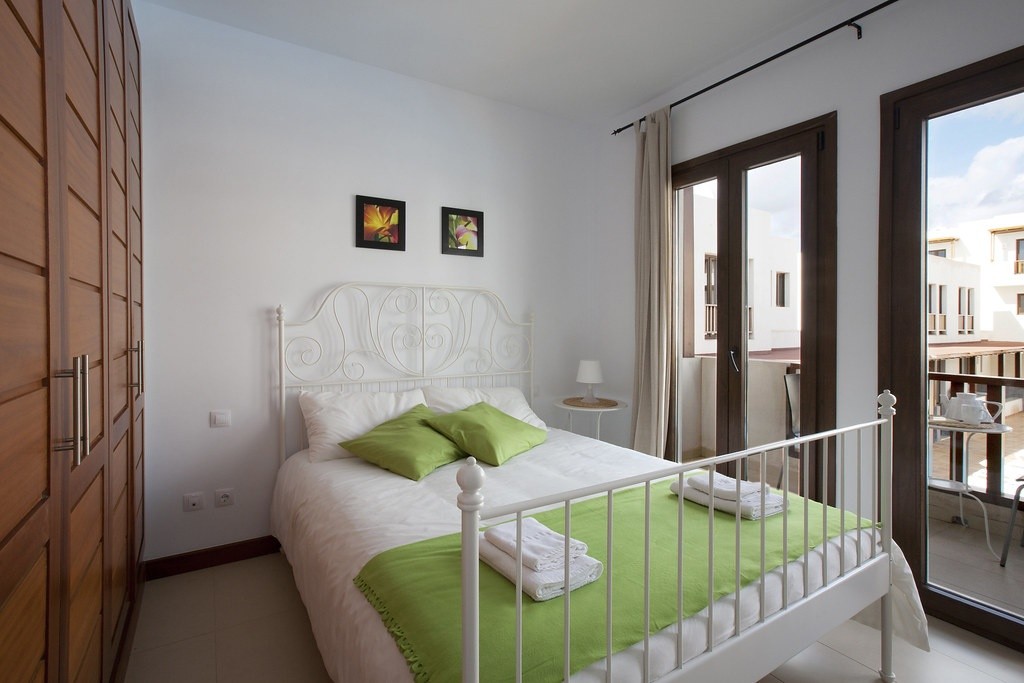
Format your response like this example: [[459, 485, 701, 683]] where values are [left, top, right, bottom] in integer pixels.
[[577, 359, 604, 404]]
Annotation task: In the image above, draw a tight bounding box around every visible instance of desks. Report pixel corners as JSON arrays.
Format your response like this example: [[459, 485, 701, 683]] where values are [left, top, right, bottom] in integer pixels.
[[553, 395, 628, 441], [928, 415, 1012, 561]]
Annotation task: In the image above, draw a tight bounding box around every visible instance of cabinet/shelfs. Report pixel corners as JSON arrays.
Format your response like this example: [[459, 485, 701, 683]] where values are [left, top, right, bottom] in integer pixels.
[[0, 0, 146, 683]]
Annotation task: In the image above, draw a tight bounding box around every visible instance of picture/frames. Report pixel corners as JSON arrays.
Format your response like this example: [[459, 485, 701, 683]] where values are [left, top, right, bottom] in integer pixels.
[[442, 206, 484, 258], [355, 195, 406, 251]]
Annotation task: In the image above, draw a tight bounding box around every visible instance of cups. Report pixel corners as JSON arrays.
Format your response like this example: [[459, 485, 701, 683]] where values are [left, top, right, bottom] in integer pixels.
[[960, 404, 987, 425]]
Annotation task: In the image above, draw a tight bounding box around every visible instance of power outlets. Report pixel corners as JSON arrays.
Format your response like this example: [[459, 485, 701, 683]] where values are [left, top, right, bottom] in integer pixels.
[[183, 492, 206, 511], [214, 488, 235, 508]]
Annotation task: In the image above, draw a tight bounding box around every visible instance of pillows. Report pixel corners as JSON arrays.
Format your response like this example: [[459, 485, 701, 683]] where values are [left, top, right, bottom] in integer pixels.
[[422, 386, 548, 432], [337, 403, 468, 481], [421, 401, 549, 467], [298, 388, 428, 463]]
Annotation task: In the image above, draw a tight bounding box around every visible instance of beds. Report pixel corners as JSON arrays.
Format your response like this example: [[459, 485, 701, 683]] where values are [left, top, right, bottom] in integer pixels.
[[268, 282, 929, 683]]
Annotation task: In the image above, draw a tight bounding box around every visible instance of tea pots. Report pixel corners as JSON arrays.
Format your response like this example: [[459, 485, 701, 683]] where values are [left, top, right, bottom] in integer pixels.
[[939, 393, 1003, 423]]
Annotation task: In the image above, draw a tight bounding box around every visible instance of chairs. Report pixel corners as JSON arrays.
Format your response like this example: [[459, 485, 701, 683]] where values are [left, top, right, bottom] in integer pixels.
[[776, 374, 802, 495], [999, 475, 1024, 567]]
